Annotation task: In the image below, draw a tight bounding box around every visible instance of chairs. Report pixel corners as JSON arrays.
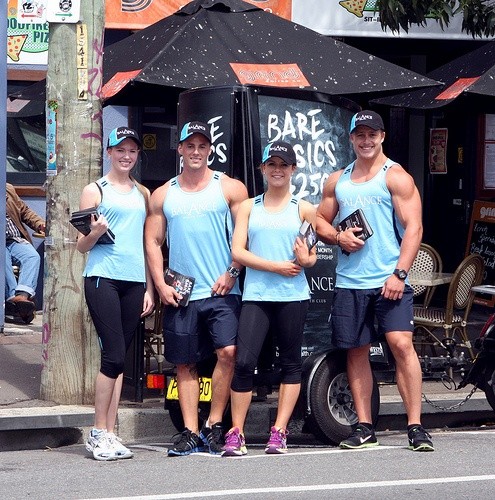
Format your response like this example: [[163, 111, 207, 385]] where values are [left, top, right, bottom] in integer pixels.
[[389, 242, 485, 384]]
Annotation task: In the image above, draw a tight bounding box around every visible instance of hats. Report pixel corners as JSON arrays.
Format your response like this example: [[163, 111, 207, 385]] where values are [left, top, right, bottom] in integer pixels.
[[261, 140, 296, 164], [179, 121, 211, 143], [107, 126, 140, 148], [349, 110, 385, 134]]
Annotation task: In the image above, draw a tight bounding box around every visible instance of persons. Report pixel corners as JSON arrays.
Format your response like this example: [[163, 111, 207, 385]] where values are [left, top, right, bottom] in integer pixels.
[[6, 110, 435, 460]]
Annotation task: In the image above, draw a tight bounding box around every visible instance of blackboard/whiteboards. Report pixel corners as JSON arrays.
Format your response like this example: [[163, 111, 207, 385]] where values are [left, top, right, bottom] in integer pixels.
[[465, 200, 495, 308]]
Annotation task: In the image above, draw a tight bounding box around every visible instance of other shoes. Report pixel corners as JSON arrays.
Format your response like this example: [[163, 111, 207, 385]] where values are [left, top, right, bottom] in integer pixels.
[[6, 294, 36, 324]]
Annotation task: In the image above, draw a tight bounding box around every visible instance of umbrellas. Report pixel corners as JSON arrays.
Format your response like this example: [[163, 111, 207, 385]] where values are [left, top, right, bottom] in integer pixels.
[[8, 0, 495, 109]]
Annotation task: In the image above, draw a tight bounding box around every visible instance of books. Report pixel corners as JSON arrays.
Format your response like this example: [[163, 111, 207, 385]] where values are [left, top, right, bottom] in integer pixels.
[[72, 212, 116, 239], [164, 267, 195, 306], [73, 207, 100, 217], [67, 213, 115, 245], [337, 208, 375, 256], [298, 220, 318, 252]]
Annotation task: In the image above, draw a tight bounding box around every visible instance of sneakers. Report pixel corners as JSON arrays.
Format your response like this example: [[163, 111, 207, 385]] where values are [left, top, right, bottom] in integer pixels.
[[199, 418, 226, 455], [220, 427, 248, 456], [167, 426, 205, 456], [264, 426, 289, 454], [407, 425, 434, 452], [338, 424, 379, 449], [85, 428, 134, 461]]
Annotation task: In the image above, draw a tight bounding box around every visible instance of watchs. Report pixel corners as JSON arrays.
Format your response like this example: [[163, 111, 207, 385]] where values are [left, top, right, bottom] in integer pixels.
[[227, 267, 240, 279], [392, 269, 407, 280]]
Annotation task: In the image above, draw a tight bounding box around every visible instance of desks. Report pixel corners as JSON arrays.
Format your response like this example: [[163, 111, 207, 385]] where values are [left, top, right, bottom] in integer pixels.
[[405, 269, 455, 286]]
